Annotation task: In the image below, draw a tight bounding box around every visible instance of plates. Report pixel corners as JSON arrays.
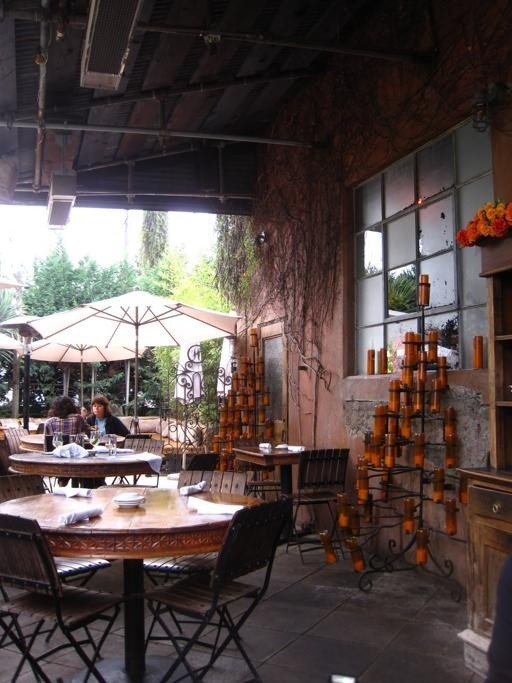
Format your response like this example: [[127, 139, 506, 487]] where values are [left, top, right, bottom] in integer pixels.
[[112, 493, 145, 509]]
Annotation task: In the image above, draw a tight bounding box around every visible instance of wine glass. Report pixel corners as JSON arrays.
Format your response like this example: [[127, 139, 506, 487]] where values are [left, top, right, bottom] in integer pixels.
[[105, 434, 117, 456]]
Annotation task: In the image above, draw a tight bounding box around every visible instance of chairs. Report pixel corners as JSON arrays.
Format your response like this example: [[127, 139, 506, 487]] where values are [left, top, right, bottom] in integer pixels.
[[0, 412, 352, 682]]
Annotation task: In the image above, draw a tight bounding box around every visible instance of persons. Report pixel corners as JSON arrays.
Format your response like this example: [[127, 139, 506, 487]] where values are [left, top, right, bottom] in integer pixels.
[[43, 395, 131, 489]]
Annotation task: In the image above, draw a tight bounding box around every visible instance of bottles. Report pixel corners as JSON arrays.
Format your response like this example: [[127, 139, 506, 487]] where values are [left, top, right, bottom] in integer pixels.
[[473, 334, 484, 368], [319, 330, 469, 569], [212, 328, 270, 471], [418, 274, 429, 304]]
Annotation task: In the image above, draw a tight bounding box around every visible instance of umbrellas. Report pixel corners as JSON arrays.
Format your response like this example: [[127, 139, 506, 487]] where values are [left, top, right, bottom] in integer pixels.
[[0, 285, 243, 432]]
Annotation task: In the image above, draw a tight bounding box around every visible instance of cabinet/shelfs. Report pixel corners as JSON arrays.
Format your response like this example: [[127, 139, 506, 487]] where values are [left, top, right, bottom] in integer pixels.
[[477, 236, 512, 471], [456, 466, 512, 654]]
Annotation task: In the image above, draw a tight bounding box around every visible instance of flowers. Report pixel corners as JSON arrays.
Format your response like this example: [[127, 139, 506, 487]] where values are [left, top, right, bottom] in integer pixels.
[[454, 198, 512, 247]]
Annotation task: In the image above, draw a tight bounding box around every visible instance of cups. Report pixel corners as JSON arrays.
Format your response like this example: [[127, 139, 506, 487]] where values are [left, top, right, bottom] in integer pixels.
[[90, 430, 101, 445], [52, 432, 63, 447]]
